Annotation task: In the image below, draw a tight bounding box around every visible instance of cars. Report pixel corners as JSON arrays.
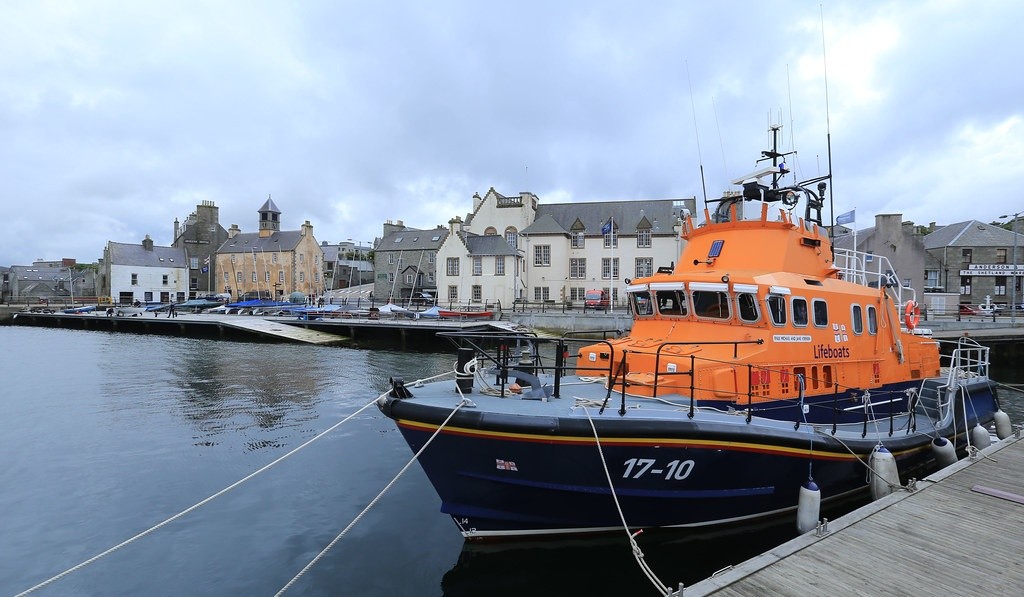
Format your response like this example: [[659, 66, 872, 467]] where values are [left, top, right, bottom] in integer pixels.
[[960, 303, 1024, 316]]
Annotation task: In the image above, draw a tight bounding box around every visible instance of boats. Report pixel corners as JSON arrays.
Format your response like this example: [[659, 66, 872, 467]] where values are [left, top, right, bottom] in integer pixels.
[[106, 307, 147, 314], [438, 310, 492, 320], [419, 305, 444, 319], [375, 3, 999, 543]]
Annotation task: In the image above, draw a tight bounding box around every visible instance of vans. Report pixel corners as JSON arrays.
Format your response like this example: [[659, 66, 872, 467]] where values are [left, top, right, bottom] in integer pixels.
[[215, 293, 232, 304], [237, 290, 272, 302]]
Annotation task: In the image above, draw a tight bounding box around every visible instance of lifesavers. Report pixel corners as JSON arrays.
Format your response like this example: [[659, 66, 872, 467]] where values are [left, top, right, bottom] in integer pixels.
[[905, 301, 920, 330]]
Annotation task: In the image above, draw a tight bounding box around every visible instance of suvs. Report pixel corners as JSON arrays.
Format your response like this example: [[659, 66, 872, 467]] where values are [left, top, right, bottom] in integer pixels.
[[410, 292, 434, 306]]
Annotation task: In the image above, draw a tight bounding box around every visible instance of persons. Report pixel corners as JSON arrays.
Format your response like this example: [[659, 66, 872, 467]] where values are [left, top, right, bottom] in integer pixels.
[[167, 300, 174, 318], [134, 299, 141, 307], [106, 308, 114, 317]]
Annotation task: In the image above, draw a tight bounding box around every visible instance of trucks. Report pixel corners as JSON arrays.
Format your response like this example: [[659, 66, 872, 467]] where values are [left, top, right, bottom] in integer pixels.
[[585, 290, 609, 309]]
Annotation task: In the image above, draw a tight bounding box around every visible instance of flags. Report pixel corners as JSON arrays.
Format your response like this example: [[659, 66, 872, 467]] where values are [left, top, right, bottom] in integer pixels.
[[200, 257, 209, 274], [836, 209, 855, 225], [601, 222, 611, 235]]
